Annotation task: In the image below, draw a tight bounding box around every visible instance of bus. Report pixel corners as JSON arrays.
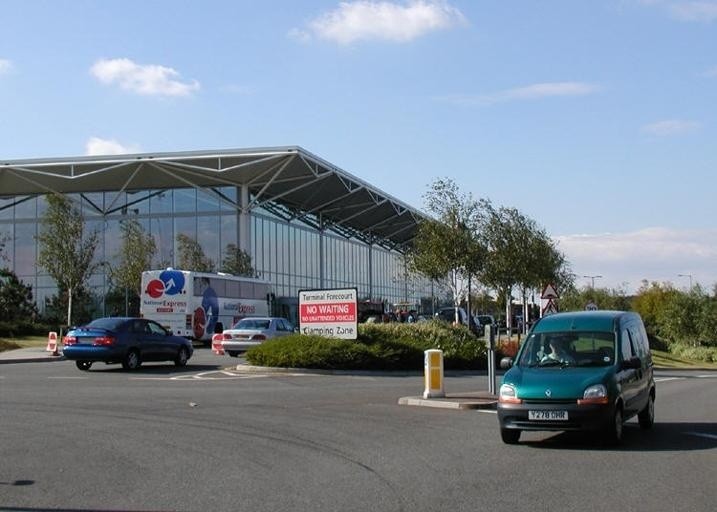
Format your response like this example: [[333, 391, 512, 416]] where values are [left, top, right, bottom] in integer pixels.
[[139, 266, 273, 346]]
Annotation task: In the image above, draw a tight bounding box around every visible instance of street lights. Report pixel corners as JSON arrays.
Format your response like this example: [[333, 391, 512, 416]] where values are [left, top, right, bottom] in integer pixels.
[[560, 273, 574, 313], [677, 273, 694, 302], [583, 275, 602, 311]]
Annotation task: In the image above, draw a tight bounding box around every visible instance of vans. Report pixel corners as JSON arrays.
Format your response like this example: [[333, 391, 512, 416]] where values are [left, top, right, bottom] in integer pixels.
[[494, 311, 659, 443]]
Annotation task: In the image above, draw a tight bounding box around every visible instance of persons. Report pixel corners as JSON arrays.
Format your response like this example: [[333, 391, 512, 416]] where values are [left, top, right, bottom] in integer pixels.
[[541, 336, 576, 365], [408, 310, 416, 323]]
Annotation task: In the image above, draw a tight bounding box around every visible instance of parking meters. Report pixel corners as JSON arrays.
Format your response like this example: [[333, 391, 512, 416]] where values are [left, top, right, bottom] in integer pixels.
[[482, 322, 498, 397]]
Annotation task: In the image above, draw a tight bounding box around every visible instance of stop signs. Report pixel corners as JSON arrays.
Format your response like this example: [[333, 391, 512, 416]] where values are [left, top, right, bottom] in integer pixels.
[[212, 334, 224, 349], [48, 332, 57, 350]]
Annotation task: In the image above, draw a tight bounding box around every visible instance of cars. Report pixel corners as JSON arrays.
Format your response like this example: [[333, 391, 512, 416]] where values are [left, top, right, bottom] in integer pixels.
[[432, 307, 533, 340], [62, 314, 196, 372], [222, 317, 297, 358]]
